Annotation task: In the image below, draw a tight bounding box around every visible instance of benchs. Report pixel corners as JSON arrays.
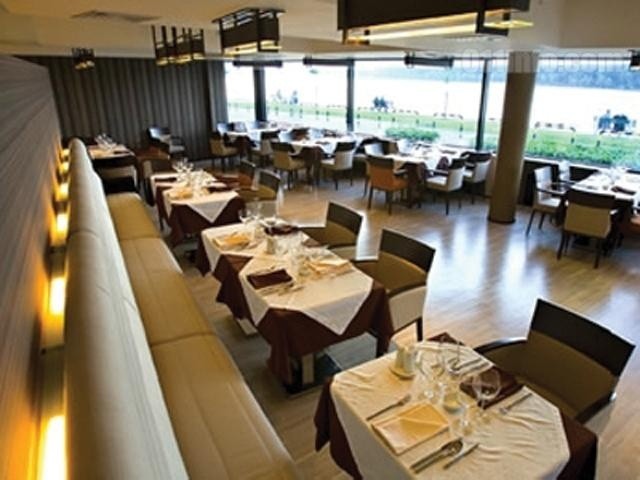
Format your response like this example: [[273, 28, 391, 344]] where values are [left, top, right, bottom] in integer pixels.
[[66, 137, 304, 480]]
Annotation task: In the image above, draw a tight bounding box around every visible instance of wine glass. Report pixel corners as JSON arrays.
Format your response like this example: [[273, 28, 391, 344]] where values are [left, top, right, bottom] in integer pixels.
[[94, 130, 117, 156], [171, 156, 194, 187], [237, 203, 319, 283], [415, 334, 502, 430], [595, 160, 626, 192]]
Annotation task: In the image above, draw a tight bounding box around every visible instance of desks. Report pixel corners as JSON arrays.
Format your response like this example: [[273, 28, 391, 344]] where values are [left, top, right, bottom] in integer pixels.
[[199, 217, 390, 397], [391, 153, 440, 200]]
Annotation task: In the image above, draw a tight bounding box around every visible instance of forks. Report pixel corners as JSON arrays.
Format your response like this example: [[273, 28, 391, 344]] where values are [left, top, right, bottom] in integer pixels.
[[365, 394, 412, 420]]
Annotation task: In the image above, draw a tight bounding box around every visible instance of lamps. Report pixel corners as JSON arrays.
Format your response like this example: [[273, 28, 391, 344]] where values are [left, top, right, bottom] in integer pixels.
[[406, 56, 455, 67], [338, 3, 532, 41], [232, 60, 282, 67], [152, 25, 204, 64], [212, 7, 285, 55], [303, 58, 355, 67]]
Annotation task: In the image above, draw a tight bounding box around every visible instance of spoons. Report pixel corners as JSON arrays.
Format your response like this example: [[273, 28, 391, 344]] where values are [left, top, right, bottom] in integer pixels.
[[416, 441, 463, 475]]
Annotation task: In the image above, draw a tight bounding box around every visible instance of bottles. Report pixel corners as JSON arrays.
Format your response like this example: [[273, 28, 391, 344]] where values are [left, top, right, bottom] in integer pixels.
[[394, 341, 417, 373]]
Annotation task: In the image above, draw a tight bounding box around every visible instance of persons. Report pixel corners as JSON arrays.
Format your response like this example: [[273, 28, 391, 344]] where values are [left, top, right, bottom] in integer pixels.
[[599, 109, 615, 143], [372, 97, 388, 113], [274, 90, 301, 112]]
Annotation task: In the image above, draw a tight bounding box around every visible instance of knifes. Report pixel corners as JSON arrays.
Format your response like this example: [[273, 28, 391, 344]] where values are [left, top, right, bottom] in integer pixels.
[[407, 433, 468, 467], [444, 442, 479, 470]]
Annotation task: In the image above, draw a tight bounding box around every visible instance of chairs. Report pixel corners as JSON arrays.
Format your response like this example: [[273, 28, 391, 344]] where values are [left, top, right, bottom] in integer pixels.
[[525, 165, 640, 268], [352, 229, 435, 353], [305, 200, 362, 260], [453, 160, 491, 202], [367, 155, 410, 213], [420, 157, 468, 214], [477, 299, 636, 426], [140, 121, 393, 236]]
